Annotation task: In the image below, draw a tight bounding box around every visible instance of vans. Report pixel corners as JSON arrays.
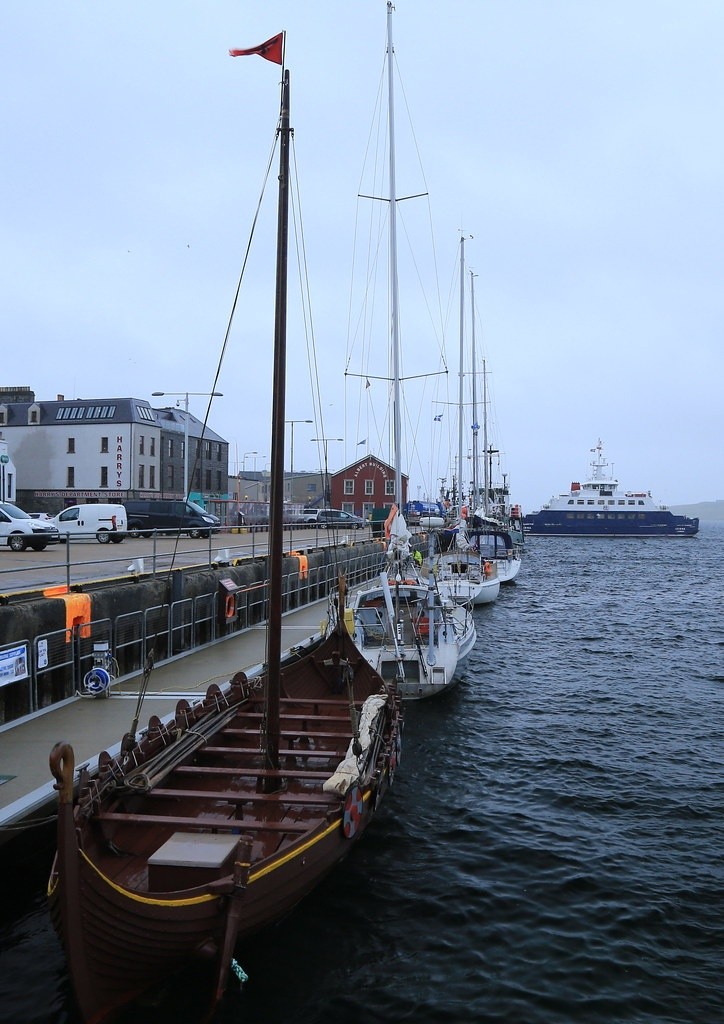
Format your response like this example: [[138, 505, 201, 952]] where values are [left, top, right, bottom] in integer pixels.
[[46, 503, 128, 545], [122, 499, 222, 539]]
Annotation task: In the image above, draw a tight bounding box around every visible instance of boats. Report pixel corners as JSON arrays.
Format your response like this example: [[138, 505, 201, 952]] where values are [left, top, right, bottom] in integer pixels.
[[521, 436, 701, 537]]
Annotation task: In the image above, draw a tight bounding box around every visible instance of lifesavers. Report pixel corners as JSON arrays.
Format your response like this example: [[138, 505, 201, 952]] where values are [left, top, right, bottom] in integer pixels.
[[83, 667, 111, 695], [484, 562, 492, 576], [225, 594, 235, 618]]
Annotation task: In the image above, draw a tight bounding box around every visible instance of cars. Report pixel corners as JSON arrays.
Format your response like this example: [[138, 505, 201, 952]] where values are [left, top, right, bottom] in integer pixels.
[[0, 500, 59, 552], [282, 508, 345, 529]]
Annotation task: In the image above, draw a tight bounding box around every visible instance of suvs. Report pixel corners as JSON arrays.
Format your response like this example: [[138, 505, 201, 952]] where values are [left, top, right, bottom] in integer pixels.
[[26, 512, 56, 522], [317, 508, 366, 530]]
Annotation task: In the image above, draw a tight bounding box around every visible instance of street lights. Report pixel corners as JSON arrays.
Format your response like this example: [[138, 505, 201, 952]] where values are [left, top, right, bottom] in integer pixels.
[[311, 439, 344, 510], [151, 392, 223, 499], [285, 419, 314, 477], [245, 455, 266, 481]]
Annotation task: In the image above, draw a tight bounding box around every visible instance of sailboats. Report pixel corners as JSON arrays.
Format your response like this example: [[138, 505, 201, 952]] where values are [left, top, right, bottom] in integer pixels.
[[321, 0, 524, 711], [48, 69, 405, 1024]]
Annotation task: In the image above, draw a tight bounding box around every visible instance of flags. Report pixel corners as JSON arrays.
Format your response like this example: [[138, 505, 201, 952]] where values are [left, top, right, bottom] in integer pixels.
[[229, 32, 283, 66]]
